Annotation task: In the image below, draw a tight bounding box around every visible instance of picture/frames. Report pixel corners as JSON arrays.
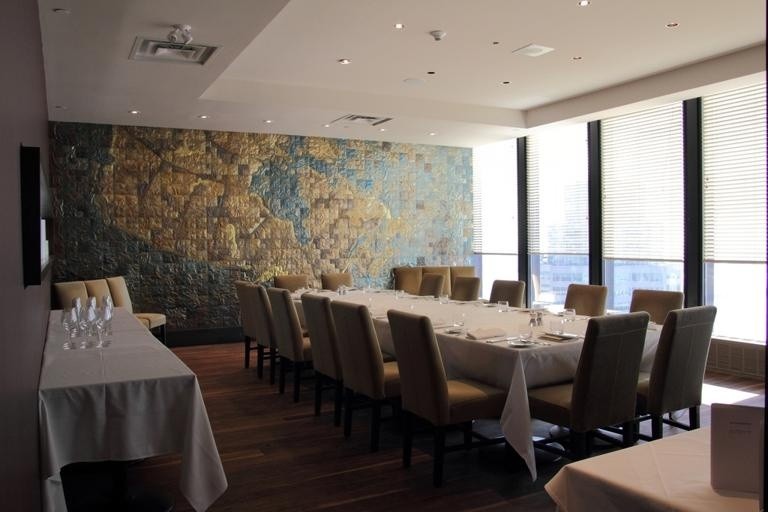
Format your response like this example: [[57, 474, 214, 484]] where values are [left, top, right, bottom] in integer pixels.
[[19, 145, 52, 290]]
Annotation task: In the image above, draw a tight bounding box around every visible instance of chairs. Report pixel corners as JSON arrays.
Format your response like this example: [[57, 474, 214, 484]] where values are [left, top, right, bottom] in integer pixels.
[[54, 280, 88, 312], [83, 278, 148, 335], [106, 275, 166, 346]]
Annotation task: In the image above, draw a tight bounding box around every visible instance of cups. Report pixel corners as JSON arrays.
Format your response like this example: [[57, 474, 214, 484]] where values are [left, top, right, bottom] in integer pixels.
[[525, 306, 577, 336], [293, 279, 509, 335]]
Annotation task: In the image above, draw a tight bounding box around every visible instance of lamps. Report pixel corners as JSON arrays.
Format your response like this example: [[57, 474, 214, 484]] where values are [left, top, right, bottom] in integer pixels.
[[167, 22, 194, 44]]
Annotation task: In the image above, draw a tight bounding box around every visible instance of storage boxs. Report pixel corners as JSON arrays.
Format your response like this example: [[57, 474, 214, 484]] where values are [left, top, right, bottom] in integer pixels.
[[707, 396, 766, 501]]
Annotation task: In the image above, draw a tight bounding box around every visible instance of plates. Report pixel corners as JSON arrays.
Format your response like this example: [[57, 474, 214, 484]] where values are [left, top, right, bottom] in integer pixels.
[[507, 339, 536, 347]]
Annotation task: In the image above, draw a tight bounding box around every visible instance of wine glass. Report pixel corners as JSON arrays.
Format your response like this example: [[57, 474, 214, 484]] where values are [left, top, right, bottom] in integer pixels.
[[61, 294, 114, 352]]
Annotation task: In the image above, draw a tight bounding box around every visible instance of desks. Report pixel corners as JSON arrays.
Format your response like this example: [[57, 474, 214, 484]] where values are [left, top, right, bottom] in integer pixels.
[[562, 422, 763, 512], [38, 308, 227, 510]]
